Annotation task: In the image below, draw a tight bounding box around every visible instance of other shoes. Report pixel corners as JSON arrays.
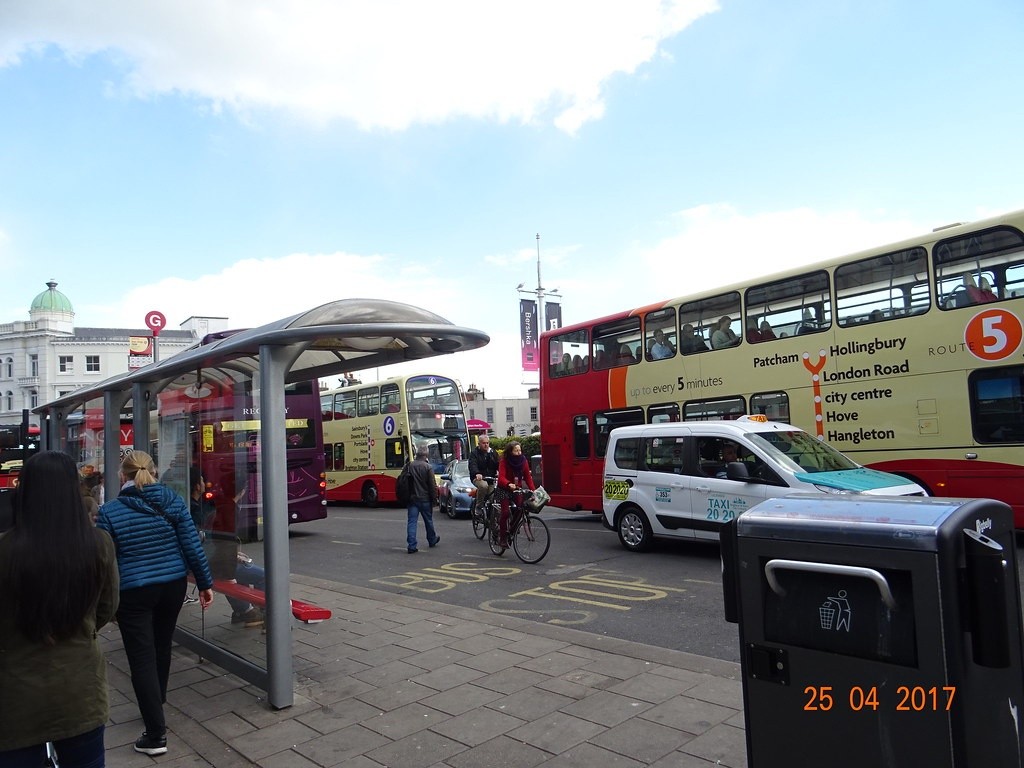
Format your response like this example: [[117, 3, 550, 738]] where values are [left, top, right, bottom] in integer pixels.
[[408, 548, 418, 553], [475, 506, 483, 515], [500, 539, 509, 549], [230, 610, 242, 622], [430, 536, 440, 547]]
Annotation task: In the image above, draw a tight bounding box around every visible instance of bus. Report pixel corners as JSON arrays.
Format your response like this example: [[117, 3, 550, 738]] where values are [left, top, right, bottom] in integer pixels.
[[320, 372, 472, 508], [539, 208, 1023, 531], [1, 378, 327, 539]]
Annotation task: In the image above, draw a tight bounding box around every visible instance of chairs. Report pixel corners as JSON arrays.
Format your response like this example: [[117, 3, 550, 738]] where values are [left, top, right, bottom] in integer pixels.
[[551, 273, 997, 375]]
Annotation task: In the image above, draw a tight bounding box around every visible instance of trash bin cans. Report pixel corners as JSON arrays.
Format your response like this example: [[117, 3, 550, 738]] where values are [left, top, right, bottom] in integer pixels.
[[720, 493, 1024, 768]]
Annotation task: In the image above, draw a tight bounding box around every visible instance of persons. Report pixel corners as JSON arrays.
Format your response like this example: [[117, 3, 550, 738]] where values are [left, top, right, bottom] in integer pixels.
[[0, 450, 121, 768], [557, 315, 740, 376], [95, 451, 214, 755], [497, 441, 535, 546], [402, 446, 441, 554], [469, 434, 501, 545], [76, 459, 272, 634], [713, 444, 762, 479]]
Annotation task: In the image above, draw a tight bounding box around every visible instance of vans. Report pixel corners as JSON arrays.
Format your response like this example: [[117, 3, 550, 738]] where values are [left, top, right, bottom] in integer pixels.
[[600, 414, 932, 553]]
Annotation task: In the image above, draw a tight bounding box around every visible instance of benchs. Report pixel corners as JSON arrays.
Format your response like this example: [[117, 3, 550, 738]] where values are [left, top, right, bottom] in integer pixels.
[[183, 571, 332, 619]]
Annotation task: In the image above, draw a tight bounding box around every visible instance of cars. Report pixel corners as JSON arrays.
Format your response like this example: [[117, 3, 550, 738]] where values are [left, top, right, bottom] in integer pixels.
[[438, 459, 478, 518]]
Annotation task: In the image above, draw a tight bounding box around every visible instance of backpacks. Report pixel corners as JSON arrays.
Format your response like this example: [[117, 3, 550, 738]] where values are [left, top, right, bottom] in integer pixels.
[[396, 462, 412, 501]]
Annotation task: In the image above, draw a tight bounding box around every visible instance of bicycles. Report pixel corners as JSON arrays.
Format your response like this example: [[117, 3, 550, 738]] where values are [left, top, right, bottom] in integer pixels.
[[471, 477, 551, 565]]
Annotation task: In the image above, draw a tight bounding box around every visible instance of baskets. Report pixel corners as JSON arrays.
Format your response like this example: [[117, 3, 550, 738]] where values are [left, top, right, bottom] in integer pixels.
[[525, 490, 549, 513]]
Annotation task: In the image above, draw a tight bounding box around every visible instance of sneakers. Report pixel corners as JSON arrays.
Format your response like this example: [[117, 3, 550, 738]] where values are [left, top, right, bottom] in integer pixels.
[[135, 730, 167, 755], [244, 610, 264, 626]]
